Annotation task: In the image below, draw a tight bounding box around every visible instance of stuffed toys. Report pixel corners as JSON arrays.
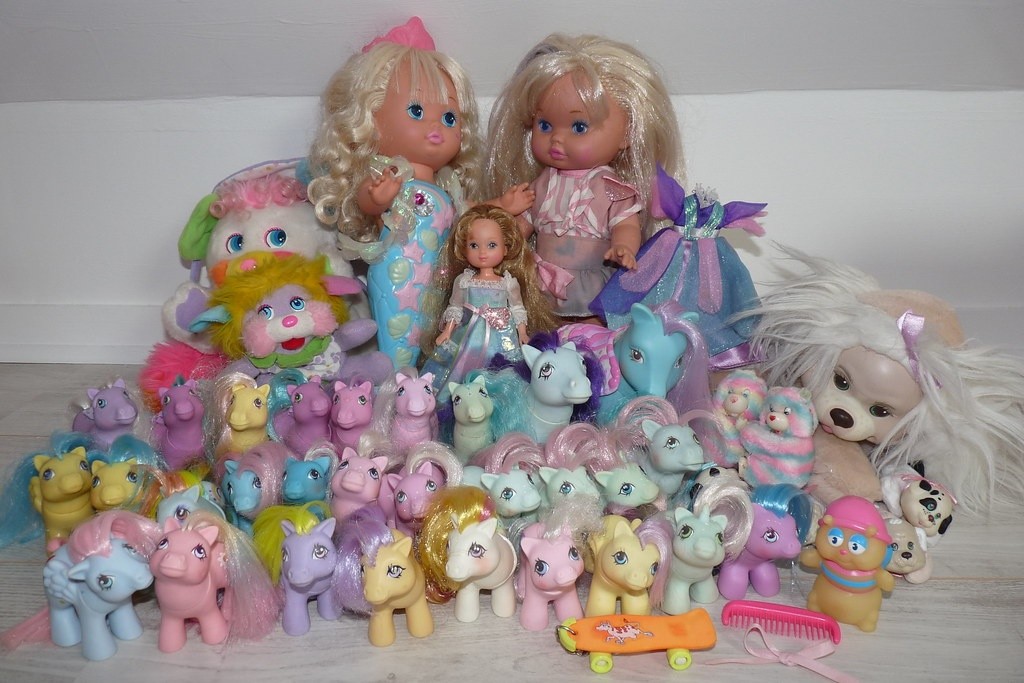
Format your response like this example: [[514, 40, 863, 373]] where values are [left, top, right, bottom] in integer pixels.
[[188, 252, 395, 388], [162, 157, 379, 356], [728, 240, 1024, 510]]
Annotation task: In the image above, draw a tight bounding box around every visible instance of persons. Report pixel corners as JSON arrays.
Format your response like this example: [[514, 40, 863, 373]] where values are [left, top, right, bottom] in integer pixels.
[[420, 204, 559, 405], [305, 15, 536, 366], [487, 32, 685, 326]]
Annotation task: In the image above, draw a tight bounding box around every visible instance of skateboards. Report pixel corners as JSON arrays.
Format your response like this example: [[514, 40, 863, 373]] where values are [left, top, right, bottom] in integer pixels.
[[560, 608, 716, 672]]
